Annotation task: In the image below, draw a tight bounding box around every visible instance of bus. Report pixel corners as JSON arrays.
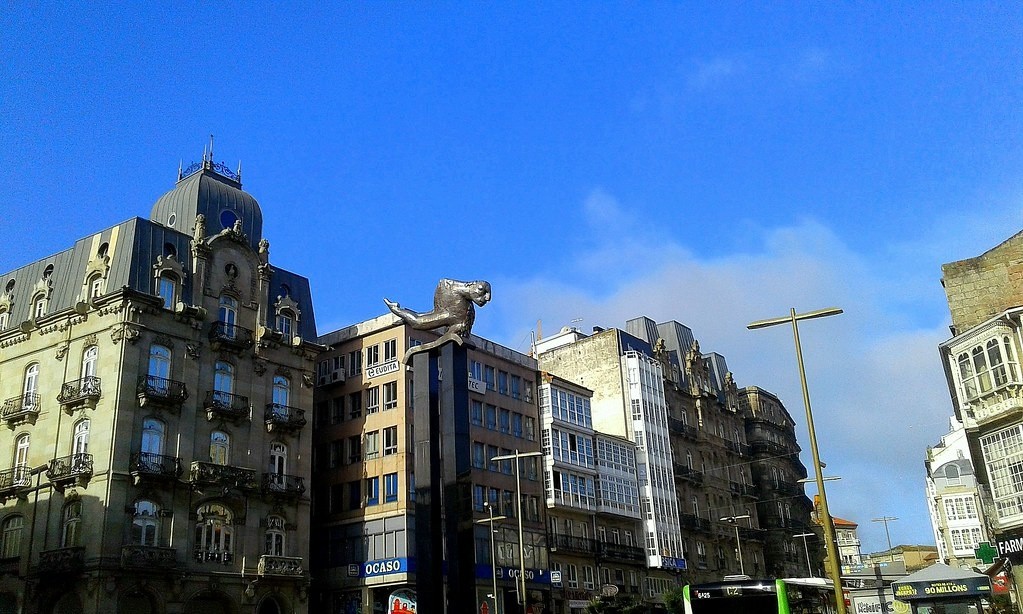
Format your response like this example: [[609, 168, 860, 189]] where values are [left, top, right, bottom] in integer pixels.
[[681, 574, 841, 614]]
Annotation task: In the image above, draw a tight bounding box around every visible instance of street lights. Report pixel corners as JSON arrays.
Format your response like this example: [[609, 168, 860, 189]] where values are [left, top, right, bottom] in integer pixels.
[[869, 517, 899, 561], [791, 532, 818, 578], [490, 448, 544, 614], [475, 500, 514, 614], [718, 515, 752, 575]]
